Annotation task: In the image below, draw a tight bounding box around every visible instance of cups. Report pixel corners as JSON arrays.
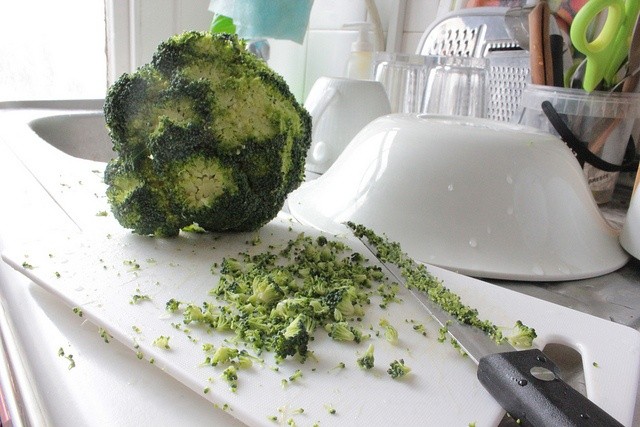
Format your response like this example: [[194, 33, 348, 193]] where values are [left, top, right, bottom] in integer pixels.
[[298, 75, 393, 175], [372, 49, 492, 118]]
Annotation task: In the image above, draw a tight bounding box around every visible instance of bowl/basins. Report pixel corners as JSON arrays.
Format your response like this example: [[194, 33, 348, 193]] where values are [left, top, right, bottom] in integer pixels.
[[287, 112, 630, 273]]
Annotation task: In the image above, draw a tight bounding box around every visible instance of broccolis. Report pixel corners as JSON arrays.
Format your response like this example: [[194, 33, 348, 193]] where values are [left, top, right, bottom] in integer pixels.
[[103, 31, 311, 236]]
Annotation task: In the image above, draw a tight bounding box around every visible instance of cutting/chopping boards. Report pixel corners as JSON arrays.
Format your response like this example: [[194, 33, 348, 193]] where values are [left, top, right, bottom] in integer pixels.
[[1, 225, 637, 427]]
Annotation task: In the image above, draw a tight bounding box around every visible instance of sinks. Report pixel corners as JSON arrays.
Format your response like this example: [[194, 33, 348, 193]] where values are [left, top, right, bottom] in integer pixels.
[[26, 112, 125, 165]]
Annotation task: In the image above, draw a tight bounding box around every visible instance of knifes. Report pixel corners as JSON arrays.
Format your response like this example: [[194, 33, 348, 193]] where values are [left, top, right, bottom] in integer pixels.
[[343, 219, 627, 426]]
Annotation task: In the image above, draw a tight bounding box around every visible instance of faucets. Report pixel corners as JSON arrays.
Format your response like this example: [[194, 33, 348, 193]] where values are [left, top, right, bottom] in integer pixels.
[[249, 38, 272, 61]]
